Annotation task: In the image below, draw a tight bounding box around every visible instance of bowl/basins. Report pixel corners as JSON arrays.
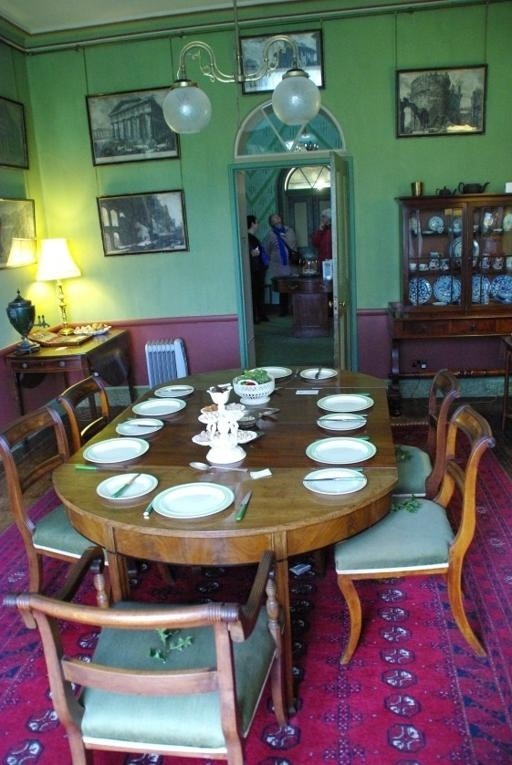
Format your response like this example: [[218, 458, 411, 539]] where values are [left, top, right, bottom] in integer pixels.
[[76, 325, 110, 336]]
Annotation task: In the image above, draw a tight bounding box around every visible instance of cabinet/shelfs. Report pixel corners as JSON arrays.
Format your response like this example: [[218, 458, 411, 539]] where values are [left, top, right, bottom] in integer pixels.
[[388, 191, 512, 315]]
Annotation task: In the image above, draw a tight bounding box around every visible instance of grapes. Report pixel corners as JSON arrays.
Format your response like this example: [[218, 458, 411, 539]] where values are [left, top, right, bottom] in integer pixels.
[[237, 368, 270, 384]]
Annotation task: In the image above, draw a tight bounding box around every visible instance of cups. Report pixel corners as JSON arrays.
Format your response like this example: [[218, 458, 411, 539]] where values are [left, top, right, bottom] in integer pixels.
[[408, 211, 511, 273], [410, 180, 424, 197]]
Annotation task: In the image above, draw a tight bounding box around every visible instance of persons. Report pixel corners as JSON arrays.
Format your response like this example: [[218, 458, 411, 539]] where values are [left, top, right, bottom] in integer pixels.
[[246, 214, 267, 325], [261, 213, 299, 318], [311, 207, 331, 262]]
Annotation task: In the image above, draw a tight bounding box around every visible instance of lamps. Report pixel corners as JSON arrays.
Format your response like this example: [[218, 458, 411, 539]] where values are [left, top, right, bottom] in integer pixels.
[[37, 237, 82, 335], [162, 0, 320, 134], [6, 237, 35, 267]]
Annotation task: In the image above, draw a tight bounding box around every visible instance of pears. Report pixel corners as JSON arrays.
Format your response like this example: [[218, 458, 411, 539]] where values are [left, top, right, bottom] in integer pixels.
[[92, 323, 108, 331]]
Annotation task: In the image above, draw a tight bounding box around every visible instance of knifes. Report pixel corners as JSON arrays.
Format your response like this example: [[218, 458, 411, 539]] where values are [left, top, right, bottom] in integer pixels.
[[237, 488, 253, 522]]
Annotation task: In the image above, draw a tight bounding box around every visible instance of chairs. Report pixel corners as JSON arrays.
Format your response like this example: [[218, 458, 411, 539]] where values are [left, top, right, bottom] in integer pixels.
[[380, 367, 462, 499], [0, 406, 144, 592], [16, 545, 293, 765], [333, 402, 494, 665], [56, 372, 112, 452]]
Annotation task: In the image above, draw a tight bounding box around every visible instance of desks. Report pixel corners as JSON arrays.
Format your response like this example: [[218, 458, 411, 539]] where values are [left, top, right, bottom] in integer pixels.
[[269, 274, 333, 339], [385, 309, 512, 416], [501, 335, 512, 426]]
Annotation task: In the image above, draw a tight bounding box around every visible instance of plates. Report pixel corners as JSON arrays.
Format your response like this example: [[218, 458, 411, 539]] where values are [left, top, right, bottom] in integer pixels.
[[409, 275, 510, 305], [248, 365, 293, 381], [300, 368, 375, 495], [82, 383, 244, 522]]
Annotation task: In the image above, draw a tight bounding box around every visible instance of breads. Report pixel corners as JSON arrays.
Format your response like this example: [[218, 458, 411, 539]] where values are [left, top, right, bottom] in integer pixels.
[[205, 404, 217, 411]]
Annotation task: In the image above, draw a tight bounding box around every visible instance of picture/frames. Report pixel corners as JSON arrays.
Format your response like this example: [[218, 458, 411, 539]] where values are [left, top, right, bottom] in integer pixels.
[[0, 197, 37, 270], [239, 27, 324, 95], [0, 95, 29, 169], [96, 189, 190, 257], [395, 64, 488, 138], [85, 84, 182, 166], [322, 260, 332, 280]]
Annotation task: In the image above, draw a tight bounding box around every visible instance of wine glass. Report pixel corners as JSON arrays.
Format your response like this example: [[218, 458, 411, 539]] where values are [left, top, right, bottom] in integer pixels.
[[7, 287, 40, 353]]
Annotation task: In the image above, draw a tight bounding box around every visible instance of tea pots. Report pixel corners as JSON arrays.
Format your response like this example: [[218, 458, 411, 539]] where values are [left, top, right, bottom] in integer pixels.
[[435, 184, 458, 194], [460, 182, 490, 194]]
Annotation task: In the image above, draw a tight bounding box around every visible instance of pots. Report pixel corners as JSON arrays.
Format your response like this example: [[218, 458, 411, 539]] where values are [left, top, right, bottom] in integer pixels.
[[298, 257, 320, 277]]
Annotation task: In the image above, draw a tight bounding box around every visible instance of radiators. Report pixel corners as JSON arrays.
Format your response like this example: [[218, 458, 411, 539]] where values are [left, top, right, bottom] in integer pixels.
[[145, 337, 188, 389]]
